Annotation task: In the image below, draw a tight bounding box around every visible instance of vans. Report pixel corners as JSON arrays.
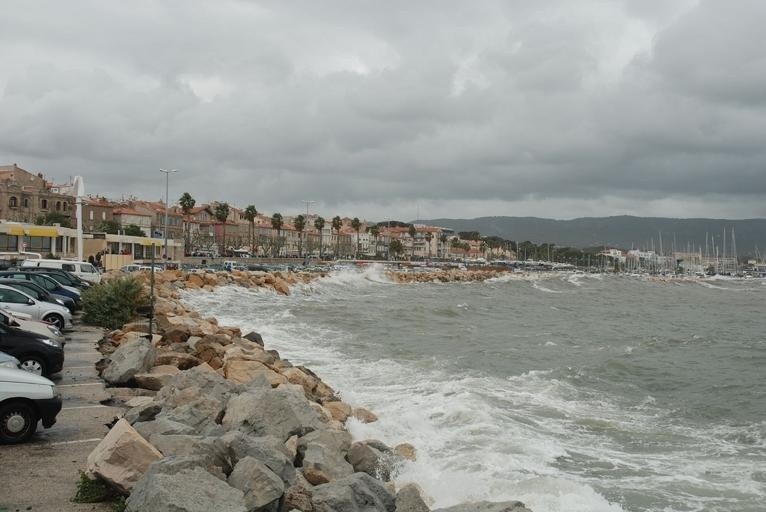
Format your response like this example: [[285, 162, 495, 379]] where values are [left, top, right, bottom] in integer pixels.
[[19, 255, 99, 281]]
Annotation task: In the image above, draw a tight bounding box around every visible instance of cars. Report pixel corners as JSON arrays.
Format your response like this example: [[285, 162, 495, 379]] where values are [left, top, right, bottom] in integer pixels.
[[0, 267, 91, 443]]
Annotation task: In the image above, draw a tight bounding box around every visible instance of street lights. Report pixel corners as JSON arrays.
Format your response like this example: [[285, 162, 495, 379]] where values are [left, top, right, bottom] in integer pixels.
[[159, 166, 179, 242]]
